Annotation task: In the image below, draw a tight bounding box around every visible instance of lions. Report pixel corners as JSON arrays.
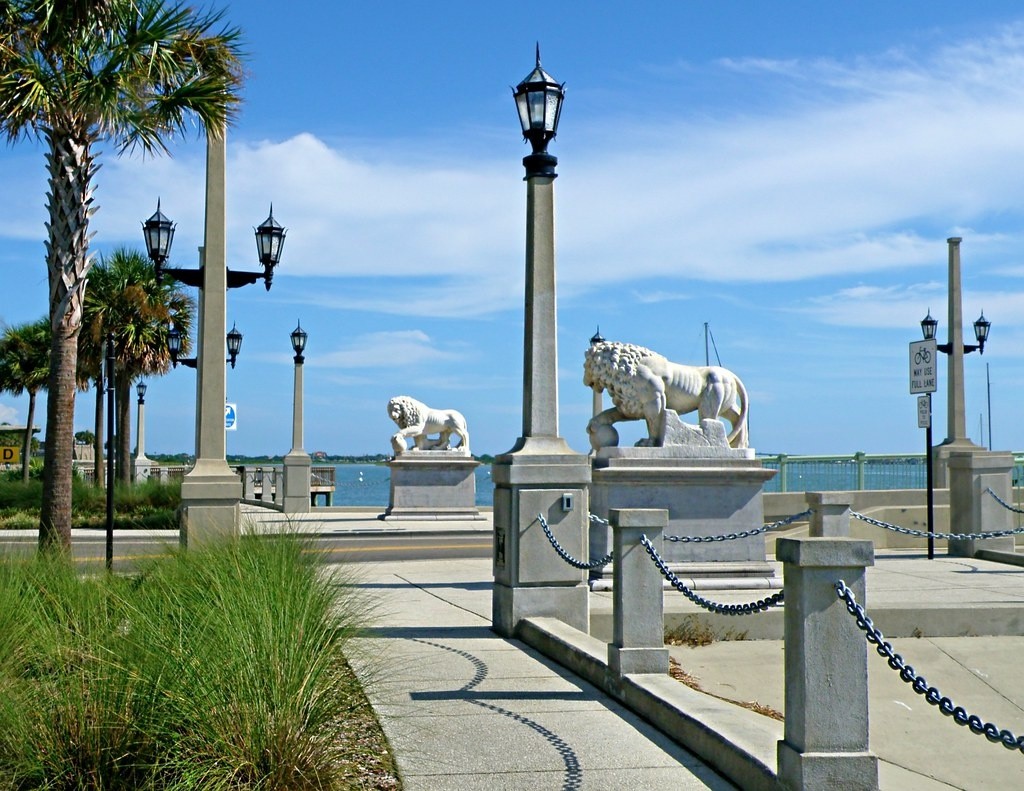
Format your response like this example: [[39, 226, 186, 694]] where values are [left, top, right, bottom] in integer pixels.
[[582, 338, 754, 447], [386, 394, 470, 453]]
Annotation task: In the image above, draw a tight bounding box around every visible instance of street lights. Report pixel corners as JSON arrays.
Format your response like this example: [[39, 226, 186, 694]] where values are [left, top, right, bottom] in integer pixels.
[[135, 373, 147, 461], [289, 316, 309, 452], [510, 39, 560, 439], [920, 237, 992, 446], [142, 49, 288, 482]]
